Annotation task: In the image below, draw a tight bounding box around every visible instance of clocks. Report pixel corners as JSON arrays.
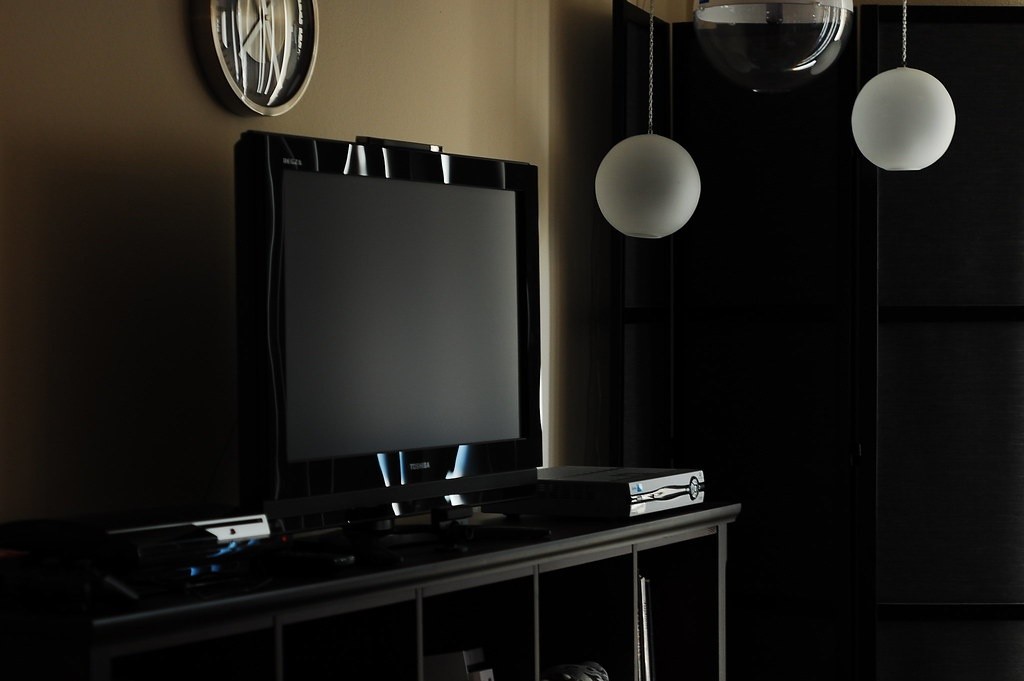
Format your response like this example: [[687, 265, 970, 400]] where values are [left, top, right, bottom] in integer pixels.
[[188, 0, 320, 119]]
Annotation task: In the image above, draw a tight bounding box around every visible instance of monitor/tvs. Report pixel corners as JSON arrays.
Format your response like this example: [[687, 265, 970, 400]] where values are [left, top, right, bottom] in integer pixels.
[[236, 129, 552, 570]]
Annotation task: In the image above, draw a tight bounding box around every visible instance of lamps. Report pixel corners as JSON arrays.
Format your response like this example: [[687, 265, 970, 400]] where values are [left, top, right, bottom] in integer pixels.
[[591, 0, 703, 241], [850, 1, 958, 173], [693, 0, 854, 95]]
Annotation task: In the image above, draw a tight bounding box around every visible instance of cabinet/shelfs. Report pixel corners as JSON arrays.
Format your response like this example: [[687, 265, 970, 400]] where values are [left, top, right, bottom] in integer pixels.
[[84, 499, 743, 681]]
